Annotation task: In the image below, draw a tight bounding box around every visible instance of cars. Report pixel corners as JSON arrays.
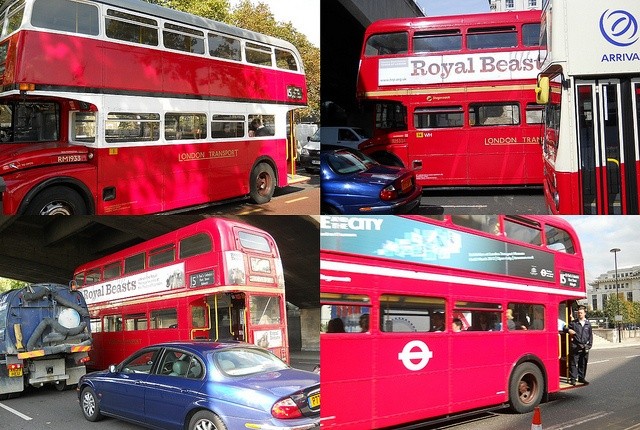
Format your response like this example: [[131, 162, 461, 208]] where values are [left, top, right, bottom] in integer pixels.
[[301, 123, 322, 176], [77, 341, 320, 429], [321, 147, 423, 216]]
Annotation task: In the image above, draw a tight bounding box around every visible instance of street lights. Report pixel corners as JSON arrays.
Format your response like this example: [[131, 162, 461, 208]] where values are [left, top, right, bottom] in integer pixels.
[[610, 248, 621, 344]]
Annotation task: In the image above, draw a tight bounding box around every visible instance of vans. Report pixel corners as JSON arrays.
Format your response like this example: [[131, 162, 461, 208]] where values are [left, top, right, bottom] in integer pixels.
[[321, 125, 370, 152]]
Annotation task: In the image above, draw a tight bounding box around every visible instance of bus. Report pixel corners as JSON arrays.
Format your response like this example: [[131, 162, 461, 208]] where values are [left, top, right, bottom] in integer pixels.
[[1, 0, 306, 217], [320, 214, 587, 430], [536, 0, 640, 213], [72, 216, 290, 370], [352, 8, 544, 193]]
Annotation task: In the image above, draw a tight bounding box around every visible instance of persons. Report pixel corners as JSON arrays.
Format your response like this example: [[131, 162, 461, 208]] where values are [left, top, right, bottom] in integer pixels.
[[528, 306, 543, 330], [489, 218, 507, 236], [489, 315, 501, 331], [325, 318, 345, 333], [359, 314, 369, 332], [428, 311, 445, 332], [506, 309, 516, 330], [478, 313, 489, 331], [563, 304, 593, 386], [251, 118, 270, 137], [452, 318, 463, 333]]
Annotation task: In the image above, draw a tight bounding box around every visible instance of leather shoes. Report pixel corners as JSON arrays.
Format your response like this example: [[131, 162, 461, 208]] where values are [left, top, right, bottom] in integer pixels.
[[578, 378, 589, 384], [571, 379, 576, 386]]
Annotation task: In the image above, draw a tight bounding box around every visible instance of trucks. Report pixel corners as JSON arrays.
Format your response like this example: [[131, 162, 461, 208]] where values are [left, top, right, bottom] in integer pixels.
[[0, 283, 92, 400]]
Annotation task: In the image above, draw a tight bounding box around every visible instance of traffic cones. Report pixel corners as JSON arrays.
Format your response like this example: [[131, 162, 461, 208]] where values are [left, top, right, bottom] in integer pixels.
[[530, 405, 543, 430]]
